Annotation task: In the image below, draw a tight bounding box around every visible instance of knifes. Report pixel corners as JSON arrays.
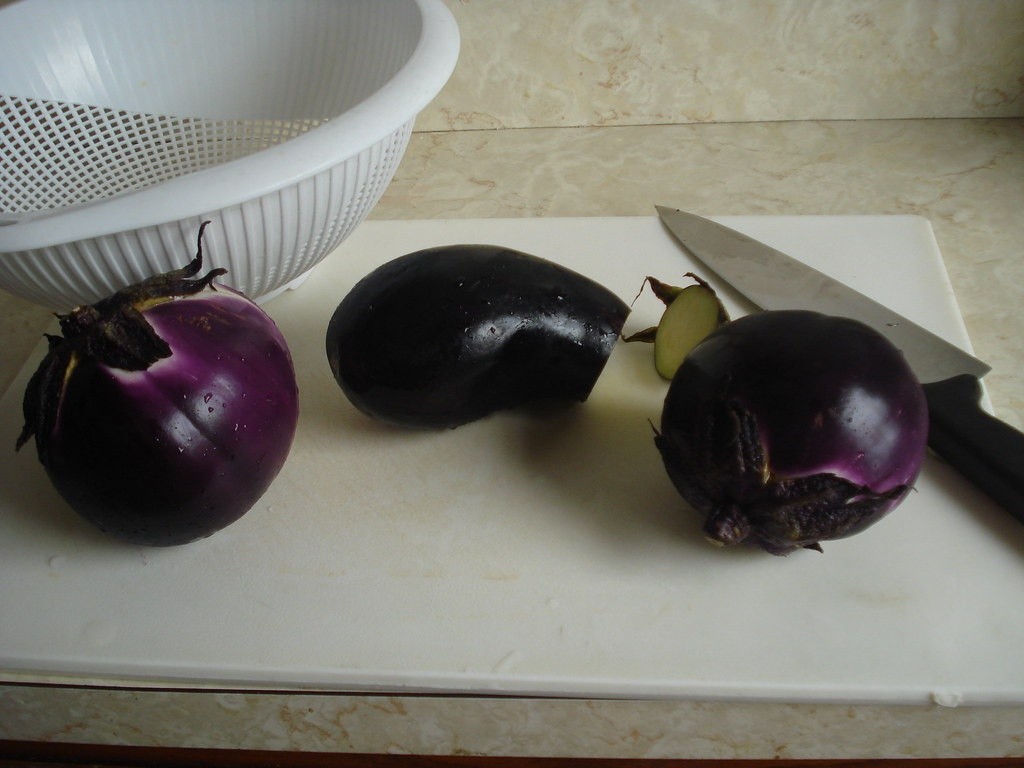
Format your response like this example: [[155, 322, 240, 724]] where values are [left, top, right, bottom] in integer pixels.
[[654, 200, 1024, 526]]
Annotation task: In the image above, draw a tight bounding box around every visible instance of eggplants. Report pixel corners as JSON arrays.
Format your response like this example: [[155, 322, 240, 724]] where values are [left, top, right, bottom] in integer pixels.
[[658, 308, 928, 552], [326, 243, 632, 427], [21, 220, 299, 547]]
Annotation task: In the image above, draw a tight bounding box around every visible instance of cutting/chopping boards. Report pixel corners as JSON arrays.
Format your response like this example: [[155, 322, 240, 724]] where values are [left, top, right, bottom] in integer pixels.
[[0, 212, 1024, 708]]
